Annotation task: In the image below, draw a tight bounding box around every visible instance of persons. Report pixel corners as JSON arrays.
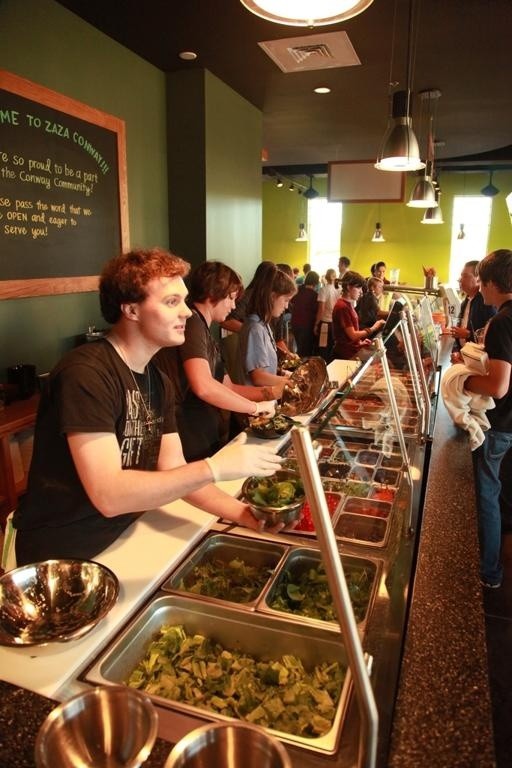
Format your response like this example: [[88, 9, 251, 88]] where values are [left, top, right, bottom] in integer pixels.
[[313, 269, 340, 358], [370, 261, 391, 284], [219, 261, 300, 362], [357, 276, 408, 338], [332, 271, 386, 359], [292, 270, 319, 357], [450, 259, 498, 366], [173, 261, 296, 467], [338, 256, 351, 281], [450, 249, 512, 589], [13, 248, 305, 567], [302, 263, 312, 273], [293, 267, 300, 275], [230, 260, 301, 400]]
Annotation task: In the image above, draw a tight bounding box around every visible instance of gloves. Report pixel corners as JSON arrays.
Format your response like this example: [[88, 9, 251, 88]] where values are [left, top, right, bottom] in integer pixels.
[[252, 399, 277, 419], [205, 432, 282, 483]]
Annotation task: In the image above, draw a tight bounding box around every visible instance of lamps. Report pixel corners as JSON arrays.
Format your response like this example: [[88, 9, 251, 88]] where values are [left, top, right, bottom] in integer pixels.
[[421, 133, 447, 227], [373, 3, 426, 172], [405, 86, 444, 211], [295, 223, 311, 242], [272, 176, 285, 190], [371, 222, 386, 244], [288, 184, 296, 193]]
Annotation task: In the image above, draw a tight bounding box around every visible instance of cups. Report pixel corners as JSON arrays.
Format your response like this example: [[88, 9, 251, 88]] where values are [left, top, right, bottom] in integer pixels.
[[389, 269, 399, 285]]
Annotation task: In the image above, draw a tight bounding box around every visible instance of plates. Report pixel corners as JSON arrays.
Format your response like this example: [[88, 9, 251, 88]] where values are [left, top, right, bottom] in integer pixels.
[[1, 558, 120, 647]]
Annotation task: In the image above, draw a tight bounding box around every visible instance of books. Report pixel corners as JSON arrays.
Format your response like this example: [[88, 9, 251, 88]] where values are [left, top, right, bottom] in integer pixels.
[[460, 341, 492, 376]]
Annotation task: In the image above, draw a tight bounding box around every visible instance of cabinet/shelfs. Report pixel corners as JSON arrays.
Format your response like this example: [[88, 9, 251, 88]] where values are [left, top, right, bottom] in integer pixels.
[[1, 289, 457, 768]]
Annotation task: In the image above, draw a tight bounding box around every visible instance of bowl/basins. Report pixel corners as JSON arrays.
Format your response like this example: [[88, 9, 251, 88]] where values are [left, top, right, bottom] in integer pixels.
[[281, 356, 330, 416], [242, 470, 307, 523], [34, 685, 159, 768], [164, 722, 294, 768], [243, 414, 295, 439]]
